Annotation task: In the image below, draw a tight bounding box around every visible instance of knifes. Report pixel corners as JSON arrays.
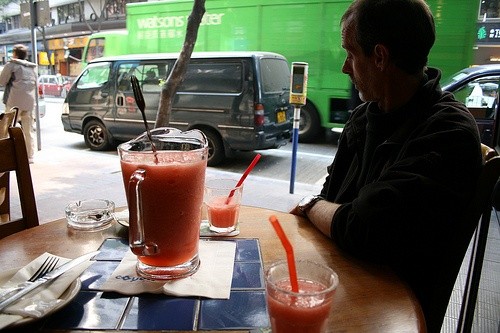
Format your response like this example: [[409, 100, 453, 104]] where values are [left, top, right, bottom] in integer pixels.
[[0, 251, 101, 311]]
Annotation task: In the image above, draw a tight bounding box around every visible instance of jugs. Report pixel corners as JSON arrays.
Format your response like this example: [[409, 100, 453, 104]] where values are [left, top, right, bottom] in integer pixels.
[[116, 126, 208, 282]]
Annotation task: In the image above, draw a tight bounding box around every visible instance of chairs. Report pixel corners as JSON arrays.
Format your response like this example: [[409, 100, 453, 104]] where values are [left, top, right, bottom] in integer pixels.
[[441, 145, 500, 333], [0, 106, 21, 222], [116, 72, 131, 90], [143, 70, 161, 84], [0, 126, 39, 241]]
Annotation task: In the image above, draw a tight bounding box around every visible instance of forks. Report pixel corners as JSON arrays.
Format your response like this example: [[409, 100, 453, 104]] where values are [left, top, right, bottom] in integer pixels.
[[0, 256, 59, 298]]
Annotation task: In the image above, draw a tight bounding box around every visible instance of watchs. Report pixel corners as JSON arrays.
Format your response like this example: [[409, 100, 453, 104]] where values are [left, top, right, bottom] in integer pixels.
[[298, 195, 324, 218]]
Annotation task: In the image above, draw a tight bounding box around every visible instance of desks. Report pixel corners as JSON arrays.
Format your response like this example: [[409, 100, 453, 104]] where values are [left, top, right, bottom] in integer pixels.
[[0, 204, 417, 333]]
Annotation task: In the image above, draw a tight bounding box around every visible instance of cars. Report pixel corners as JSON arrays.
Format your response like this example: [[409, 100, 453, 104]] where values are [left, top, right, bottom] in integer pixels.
[[440, 64, 500, 150]]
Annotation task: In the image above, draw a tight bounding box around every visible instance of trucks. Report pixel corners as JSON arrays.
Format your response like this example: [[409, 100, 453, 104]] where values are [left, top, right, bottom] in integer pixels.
[[80, 0, 481, 142]]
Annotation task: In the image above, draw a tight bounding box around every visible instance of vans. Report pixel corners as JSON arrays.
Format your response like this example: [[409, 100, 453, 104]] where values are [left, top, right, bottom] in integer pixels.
[[60, 52, 295, 167], [38, 75, 73, 98]]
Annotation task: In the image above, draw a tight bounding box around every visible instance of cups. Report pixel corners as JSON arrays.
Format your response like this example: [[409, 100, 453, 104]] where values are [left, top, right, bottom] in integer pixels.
[[204, 179, 245, 233], [263, 260, 339, 333]]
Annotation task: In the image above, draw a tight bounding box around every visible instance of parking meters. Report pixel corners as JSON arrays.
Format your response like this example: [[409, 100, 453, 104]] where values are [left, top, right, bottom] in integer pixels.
[[287, 61, 311, 194]]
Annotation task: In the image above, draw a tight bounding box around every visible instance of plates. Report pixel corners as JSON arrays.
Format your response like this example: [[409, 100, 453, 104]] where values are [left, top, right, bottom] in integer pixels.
[[0, 265, 82, 327], [117, 208, 129, 228]]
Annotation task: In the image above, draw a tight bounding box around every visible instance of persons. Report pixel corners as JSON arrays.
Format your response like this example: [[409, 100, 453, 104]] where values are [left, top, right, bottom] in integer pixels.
[[0, 44, 36, 163], [289, 0, 482, 317]]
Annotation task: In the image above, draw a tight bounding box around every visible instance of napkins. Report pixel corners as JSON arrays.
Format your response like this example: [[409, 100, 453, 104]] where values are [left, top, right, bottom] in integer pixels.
[[0, 250, 95, 318], [87, 241, 237, 300]]
